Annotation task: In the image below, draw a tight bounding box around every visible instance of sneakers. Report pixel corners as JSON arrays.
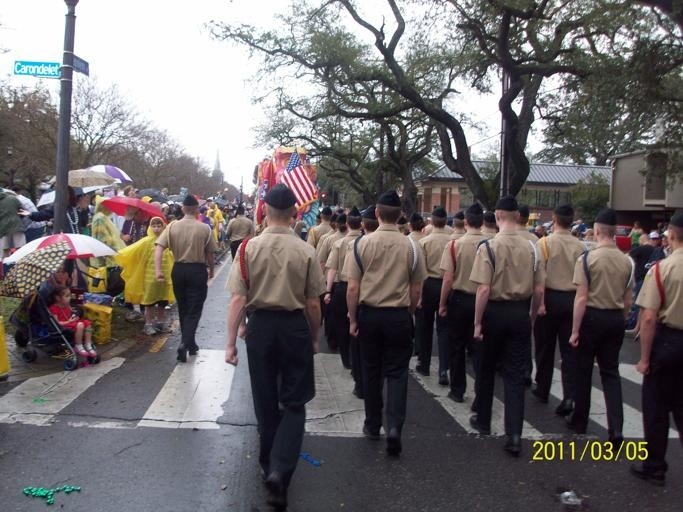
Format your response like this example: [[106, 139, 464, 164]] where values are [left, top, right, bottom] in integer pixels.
[[142, 324, 156, 336]]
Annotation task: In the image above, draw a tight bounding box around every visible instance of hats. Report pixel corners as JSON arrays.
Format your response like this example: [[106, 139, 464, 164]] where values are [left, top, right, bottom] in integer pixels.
[[398, 207, 465, 225], [361, 204, 377, 219], [555, 205, 574, 216], [483, 212, 496, 223], [263, 183, 296, 209], [466, 203, 484, 214], [518, 204, 529, 216], [594, 207, 617, 225], [72, 187, 85, 196], [670, 211, 683, 228], [316, 206, 361, 222], [183, 195, 198, 206], [493, 197, 520, 211], [648, 230, 669, 239], [376, 190, 401, 207]]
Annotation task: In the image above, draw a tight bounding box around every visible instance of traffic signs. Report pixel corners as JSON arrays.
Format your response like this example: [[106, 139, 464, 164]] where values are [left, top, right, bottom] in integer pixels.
[[14, 60, 60, 77], [74, 55, 89, 75]]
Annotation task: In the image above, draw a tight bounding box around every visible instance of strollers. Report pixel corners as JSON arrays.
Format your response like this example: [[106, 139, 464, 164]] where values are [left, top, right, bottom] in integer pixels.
[[18, 282, 101, 371]]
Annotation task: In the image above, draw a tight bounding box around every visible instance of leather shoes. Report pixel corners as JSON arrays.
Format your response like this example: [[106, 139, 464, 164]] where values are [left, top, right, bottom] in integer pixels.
[[526, 379, 549, 404], [415, 362, 464, 404], [470, 414, 492, 436], [362, 423, 380, 441], [610, 431, 623, 453], [565, 414, 586, 435], [189, 345, 200, 355], [264, 470, 288, 512], [385, 427, 401, 455], [630, 461, 666, 486], [326, 336, 365, 402], [176, 343, 187, 362], [502, 438, 522, 457], [554, 402, 573, 416]]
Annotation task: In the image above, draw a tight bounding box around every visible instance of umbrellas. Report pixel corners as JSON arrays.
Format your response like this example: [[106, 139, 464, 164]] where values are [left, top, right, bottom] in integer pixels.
[[74, 164, 132, 196], [48, 168, 116, 196]]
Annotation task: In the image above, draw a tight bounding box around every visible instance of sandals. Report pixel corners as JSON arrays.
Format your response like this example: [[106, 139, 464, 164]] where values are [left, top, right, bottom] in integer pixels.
[[84, 346, 96, 358], [73, 346, 89, 357]]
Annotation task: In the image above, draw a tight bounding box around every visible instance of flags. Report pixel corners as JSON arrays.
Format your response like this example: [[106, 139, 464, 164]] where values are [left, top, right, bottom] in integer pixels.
[[280, 147, 319, 211]]
